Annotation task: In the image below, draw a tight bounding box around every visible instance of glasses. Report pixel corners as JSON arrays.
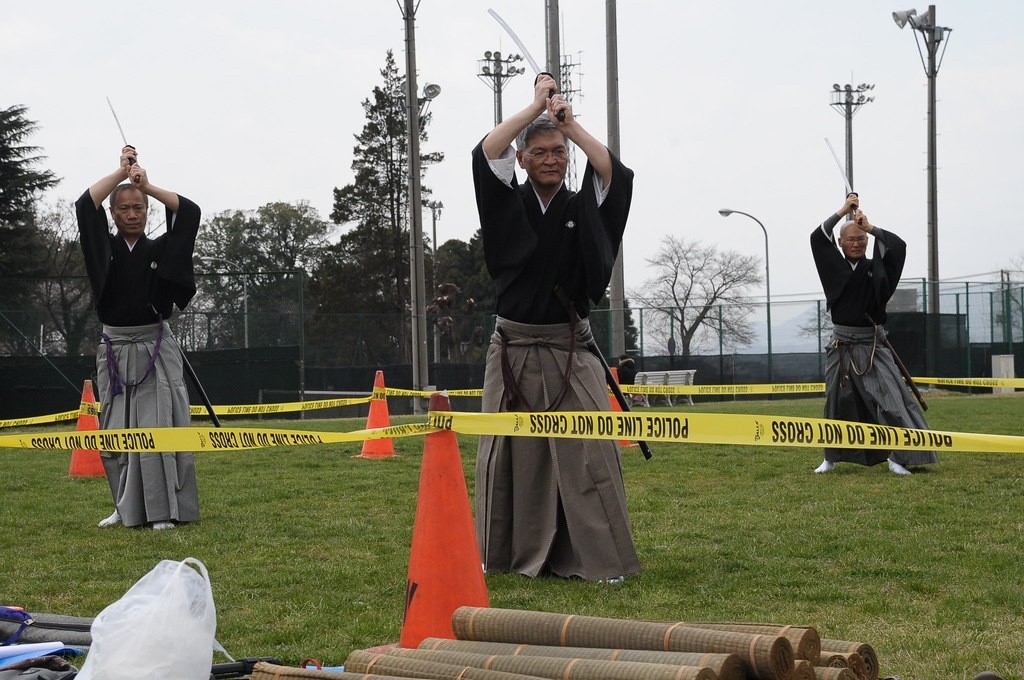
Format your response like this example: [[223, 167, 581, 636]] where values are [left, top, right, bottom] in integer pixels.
[[841, 235, 867, 244], [523, 146, 568, 160]]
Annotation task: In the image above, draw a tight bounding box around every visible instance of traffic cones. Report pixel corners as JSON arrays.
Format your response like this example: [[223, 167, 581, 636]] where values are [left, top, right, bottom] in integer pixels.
[[64, 379, 108, 479], [604, 365, 642, 447], [362, 389, 494, 656], [351, 370, 403, 460]]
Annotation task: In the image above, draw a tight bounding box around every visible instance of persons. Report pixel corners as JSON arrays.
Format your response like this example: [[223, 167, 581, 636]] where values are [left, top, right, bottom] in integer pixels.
[[74, 146, 201, 530], [809, 194, 938, 476], [474, 74, 640, 584]]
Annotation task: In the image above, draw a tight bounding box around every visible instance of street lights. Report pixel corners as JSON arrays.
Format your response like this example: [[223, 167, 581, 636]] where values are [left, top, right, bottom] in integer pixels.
[[892, 5, 942, 388], [829, 80, 874, 221], [423, 200, 448, 390], [397, 79, 441, 413], [474, 39, 525, 127], [200, 256, 250, 366], [718, 208, 774, 399]]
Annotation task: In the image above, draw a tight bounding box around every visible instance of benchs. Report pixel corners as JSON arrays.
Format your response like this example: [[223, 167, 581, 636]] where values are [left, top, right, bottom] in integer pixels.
[[624, 370, 697, 407]]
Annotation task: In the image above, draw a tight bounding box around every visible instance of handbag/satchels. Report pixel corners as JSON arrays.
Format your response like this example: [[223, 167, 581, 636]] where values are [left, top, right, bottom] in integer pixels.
[[74, 555, 216, 680]]
